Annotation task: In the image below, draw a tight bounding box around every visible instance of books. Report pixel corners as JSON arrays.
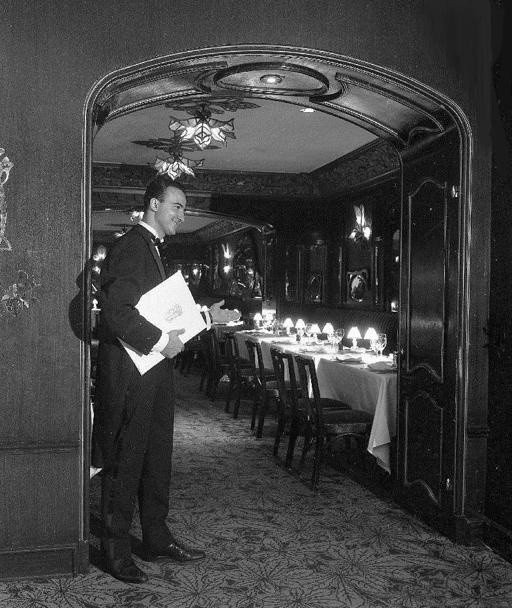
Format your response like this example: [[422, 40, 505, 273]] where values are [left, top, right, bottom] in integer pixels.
[[117, 268, 206, 375]]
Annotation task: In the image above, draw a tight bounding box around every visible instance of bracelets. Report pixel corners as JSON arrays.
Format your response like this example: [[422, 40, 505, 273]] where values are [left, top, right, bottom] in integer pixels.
[[208, 311, 214, 325]]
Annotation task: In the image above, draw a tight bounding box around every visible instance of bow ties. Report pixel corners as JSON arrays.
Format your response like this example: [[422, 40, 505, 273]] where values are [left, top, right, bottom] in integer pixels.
[[153, 238, 167, 250]]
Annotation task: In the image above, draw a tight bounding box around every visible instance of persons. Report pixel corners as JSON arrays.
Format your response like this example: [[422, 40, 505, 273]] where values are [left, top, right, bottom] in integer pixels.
[[91, 176, 245, 585]]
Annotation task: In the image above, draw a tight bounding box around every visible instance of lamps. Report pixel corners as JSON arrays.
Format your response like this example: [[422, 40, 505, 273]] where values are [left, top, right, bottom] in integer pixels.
[[132, 95, 261, 183]]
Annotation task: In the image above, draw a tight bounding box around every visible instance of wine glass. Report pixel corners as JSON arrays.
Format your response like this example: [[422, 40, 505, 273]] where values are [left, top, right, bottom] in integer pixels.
[[297, 326, 305, 342], [327, 328, 345, 353], [370, 333, 387, 359], [305, 323, 312, 342]]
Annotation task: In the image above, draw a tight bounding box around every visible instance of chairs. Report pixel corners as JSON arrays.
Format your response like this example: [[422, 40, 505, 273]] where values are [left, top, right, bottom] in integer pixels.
[[197, 328, 398, 493]]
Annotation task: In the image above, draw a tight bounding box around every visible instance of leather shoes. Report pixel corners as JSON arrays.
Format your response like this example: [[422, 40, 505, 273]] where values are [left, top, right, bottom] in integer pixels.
[[145, 542, 207, 562], [98, 553, 147, 582]]
